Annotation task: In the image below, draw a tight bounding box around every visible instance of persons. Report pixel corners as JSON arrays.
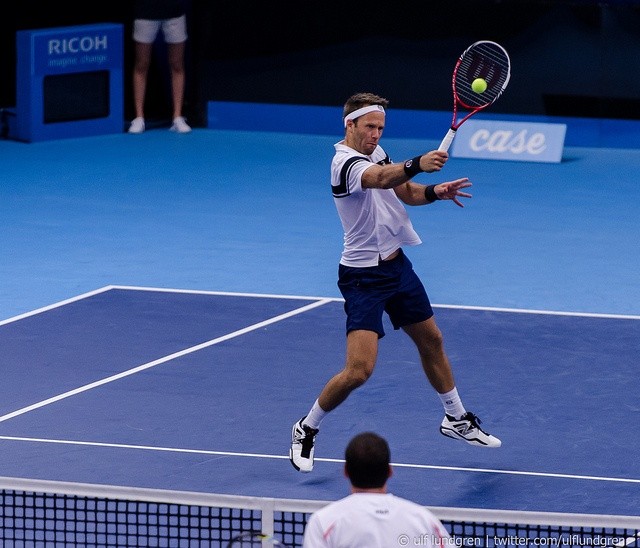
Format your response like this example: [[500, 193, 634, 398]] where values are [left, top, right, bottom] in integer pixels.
[[301, 429, 461, 548], [288, 91, 502, 474], [126, 1, 194, 134]]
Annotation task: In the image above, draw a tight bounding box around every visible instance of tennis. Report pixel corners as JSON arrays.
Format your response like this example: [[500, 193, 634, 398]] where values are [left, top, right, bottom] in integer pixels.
[[472, 78, 487, 94]]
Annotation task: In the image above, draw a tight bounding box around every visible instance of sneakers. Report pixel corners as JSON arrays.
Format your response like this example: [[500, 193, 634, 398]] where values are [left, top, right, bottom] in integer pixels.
[[440, 413, 502, 448], [289, 416, 319, 472], [171, 115, 191, 134], [128, 117, 145, 132]]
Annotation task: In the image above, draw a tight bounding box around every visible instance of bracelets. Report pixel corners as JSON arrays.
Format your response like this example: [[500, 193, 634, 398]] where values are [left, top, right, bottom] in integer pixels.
[[403, 154, 423, 178], [424, 183, 441, 202]]
